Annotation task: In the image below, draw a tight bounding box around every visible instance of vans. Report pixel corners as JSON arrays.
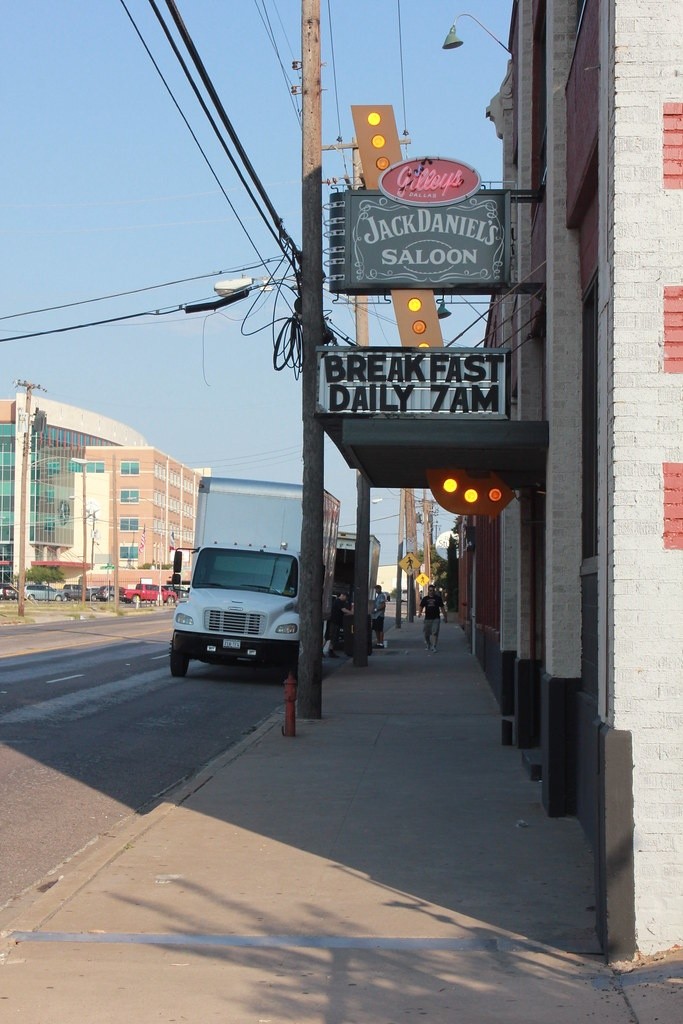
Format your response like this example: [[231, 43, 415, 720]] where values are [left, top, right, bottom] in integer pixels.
[[62, 584, 97, 602], [401, 588, 408, 603]]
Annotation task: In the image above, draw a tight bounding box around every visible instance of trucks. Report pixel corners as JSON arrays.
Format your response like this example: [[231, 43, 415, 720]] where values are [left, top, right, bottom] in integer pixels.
[[169, 476, 341, 679], [334, 529, 381, 655]]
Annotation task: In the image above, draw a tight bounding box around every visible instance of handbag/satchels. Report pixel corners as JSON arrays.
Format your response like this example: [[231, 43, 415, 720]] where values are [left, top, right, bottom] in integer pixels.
[[323, 640, 331, 658]]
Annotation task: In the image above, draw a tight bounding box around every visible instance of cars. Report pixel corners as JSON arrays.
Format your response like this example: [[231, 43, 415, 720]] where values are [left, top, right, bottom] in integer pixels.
[[0, 583, 18, 601], [22, 583, 65, 602], [86, 586, 101, 596], [162, 585, 190, 602]]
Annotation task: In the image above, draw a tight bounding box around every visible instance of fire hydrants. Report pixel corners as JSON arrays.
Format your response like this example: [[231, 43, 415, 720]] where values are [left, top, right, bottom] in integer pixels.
[[281, 669, 299, 737]]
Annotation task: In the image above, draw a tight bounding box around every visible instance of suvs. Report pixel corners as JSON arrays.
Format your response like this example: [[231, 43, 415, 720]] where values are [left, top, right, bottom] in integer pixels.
[[96, 585, 126, 602]]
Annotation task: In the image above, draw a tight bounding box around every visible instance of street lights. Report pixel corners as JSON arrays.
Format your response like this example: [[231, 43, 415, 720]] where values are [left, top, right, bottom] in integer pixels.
[[69, 495, 96, 570]]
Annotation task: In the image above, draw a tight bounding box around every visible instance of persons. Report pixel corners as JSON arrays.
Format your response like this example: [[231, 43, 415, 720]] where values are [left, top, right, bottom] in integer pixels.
[[371, 585, 386, 649], [322, 592, 353, 659], [417, 585, 448, 653]]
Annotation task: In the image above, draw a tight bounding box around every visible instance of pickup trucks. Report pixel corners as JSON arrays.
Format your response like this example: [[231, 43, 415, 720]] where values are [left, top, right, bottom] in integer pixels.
[[120, 583, 178, 605]]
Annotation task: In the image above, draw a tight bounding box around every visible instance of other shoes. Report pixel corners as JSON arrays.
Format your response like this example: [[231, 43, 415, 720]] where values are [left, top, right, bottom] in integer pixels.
[[424, 642, 431, 650], [372, 644, 384, 649], [432, 645, 437, 653]]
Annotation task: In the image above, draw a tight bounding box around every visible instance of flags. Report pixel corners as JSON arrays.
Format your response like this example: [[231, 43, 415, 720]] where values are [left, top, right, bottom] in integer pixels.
[[139, 524, 146, 553], [168, 525, 175, 552]]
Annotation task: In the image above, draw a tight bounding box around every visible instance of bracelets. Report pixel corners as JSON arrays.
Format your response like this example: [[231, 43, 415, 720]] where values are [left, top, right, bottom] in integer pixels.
[[374, 608, 378, 611]]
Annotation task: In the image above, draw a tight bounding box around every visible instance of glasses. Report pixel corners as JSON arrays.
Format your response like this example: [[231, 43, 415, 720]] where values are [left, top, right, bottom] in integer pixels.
[[429, 590, 435, 592]]
